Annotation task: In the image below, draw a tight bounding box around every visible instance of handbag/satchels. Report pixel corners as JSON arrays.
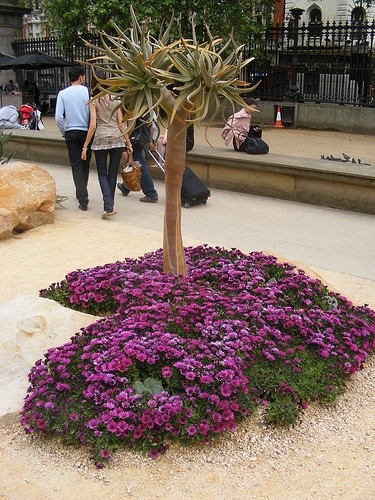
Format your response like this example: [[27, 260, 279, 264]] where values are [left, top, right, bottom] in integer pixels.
[[246, 137, 270, 155], [121, 152, 142, 192]]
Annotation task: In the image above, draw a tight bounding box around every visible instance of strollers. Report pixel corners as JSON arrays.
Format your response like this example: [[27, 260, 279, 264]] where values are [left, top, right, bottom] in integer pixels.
[[19, 102, 44, 130]]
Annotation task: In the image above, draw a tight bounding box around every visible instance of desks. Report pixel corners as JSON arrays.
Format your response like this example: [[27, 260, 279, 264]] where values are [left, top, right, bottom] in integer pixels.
[[0, 94, 35, 108]]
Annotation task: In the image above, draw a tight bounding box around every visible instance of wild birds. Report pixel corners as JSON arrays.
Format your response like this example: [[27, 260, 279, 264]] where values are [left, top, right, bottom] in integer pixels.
[[320, 153, 372, 166]]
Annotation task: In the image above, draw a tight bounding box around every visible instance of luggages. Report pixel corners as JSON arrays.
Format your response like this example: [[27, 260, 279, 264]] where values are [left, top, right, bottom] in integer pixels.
[[148, 149, 211, 208]]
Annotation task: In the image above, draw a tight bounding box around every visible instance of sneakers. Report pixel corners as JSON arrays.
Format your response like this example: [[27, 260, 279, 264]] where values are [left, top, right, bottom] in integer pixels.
[[117, 183, 130, 196], [140, 196, 158, 202]]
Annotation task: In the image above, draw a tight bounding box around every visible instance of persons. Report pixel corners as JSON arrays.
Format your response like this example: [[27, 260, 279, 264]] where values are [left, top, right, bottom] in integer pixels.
[[0, 66, 159, 218], [161, 113, 194, 153], [221, 98, 258, 152]]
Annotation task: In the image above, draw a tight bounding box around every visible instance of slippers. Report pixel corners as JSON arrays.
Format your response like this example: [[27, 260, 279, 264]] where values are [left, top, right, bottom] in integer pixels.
[[101, 211, 117, 218]]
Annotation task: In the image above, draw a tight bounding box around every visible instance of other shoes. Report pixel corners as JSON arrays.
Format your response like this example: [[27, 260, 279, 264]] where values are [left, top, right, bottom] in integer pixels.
[[78, 205, 88, 211]]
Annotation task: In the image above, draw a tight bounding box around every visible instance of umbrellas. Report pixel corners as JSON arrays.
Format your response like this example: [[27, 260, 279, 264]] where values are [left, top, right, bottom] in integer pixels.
[[0, 51, 74, 82]]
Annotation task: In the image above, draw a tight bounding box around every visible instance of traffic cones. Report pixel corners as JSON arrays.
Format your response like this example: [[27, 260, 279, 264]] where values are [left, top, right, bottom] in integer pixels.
[[272, 106, 285, 128]]
